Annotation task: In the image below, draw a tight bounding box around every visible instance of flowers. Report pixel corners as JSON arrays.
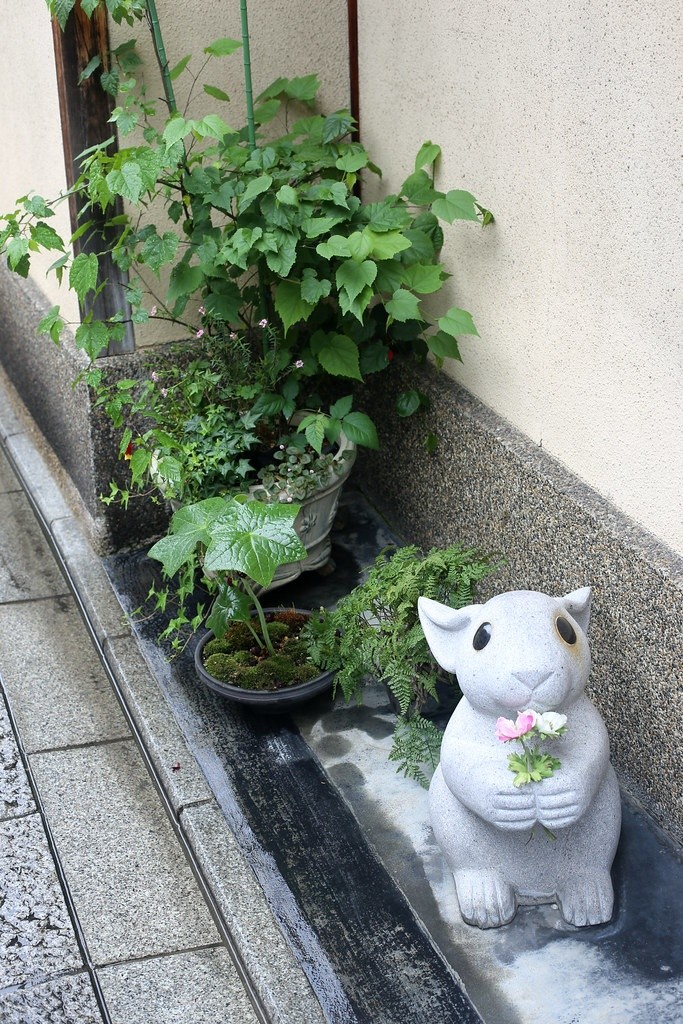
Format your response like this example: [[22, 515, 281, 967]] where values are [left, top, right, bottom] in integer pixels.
[[496, 709, 568, 846]]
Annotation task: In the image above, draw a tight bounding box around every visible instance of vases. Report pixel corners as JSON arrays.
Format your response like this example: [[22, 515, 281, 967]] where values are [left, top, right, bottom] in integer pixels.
[[146, 410, 357, 601]]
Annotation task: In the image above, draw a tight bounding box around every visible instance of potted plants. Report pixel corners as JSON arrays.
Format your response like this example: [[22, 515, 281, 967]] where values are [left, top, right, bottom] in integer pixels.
[[299, 538, 509, 791], [147, 495, 347, 708]]
